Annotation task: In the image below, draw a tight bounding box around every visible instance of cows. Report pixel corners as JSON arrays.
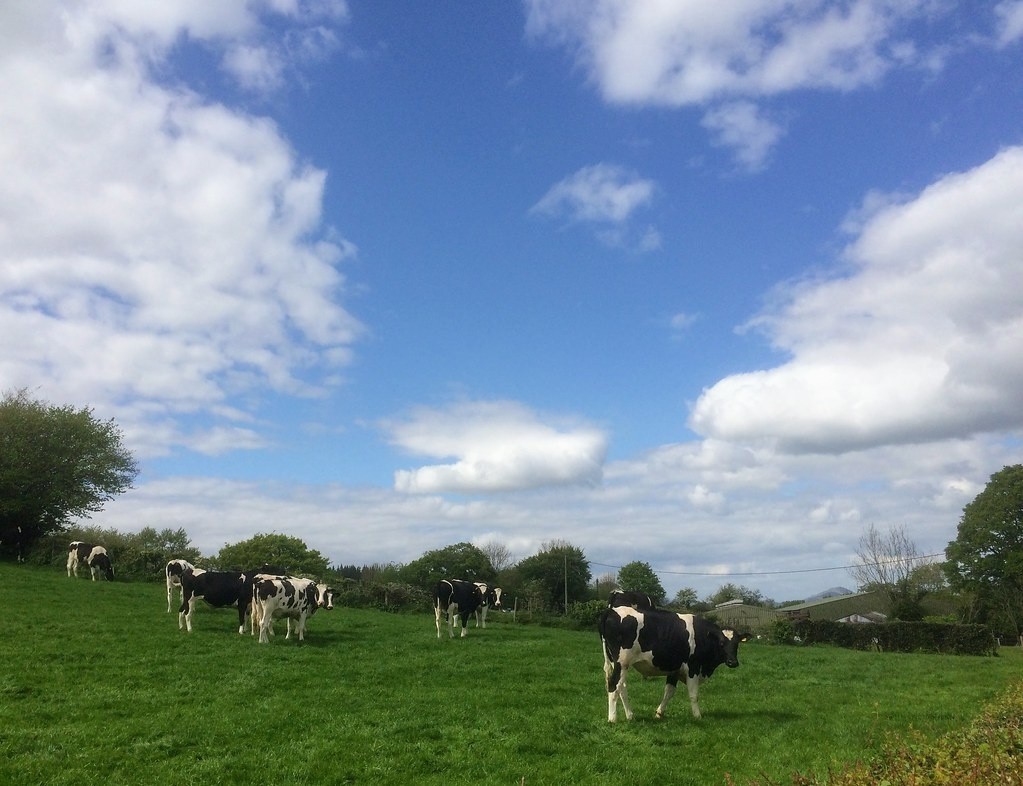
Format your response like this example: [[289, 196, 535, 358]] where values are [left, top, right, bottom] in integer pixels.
[[434, 579, 503, 638], [67, 541, 116, 582], [597, 606, 752, 723], [165, 559, 341, 643]]
